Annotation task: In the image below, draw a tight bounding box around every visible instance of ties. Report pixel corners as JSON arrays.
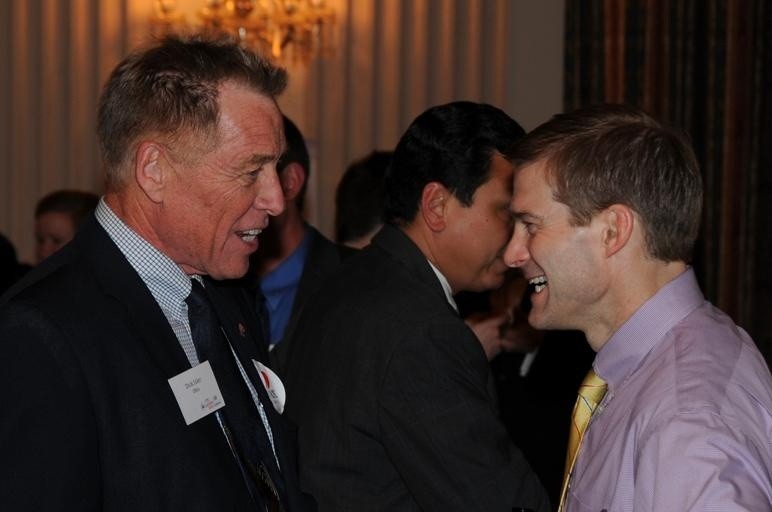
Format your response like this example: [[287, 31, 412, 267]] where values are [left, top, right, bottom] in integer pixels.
[[186, 278, 288, 512], [556, 370, 607, 512]]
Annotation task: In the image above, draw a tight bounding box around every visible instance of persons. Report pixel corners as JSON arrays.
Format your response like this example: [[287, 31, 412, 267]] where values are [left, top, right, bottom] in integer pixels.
[[503, 104, 772, 510], [243, 101, 598, 511], [2, 33, 289, 510], [2, 189, 99, 296]]
